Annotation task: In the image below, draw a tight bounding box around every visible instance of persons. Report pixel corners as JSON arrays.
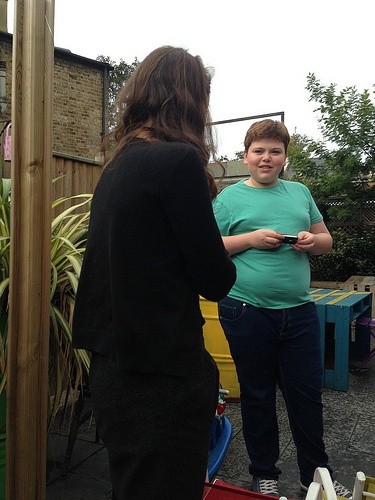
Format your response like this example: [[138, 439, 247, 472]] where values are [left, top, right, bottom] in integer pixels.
[[211, 119, 355, 500], [90, 44, 236, 500]]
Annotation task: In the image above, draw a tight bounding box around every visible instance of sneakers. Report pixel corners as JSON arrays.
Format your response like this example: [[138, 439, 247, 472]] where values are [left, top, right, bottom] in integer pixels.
[[253, 477, 280, 497], [300, 473, 353, 500]]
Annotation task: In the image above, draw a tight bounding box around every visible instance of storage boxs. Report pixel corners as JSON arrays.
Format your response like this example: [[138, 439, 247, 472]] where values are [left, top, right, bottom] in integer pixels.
[[307, 287, 374, 392], [206, 415, 232, 482]]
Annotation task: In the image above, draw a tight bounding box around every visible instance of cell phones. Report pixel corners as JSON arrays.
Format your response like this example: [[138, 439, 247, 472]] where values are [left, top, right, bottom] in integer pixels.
[[279, 235, 298, 245]]
